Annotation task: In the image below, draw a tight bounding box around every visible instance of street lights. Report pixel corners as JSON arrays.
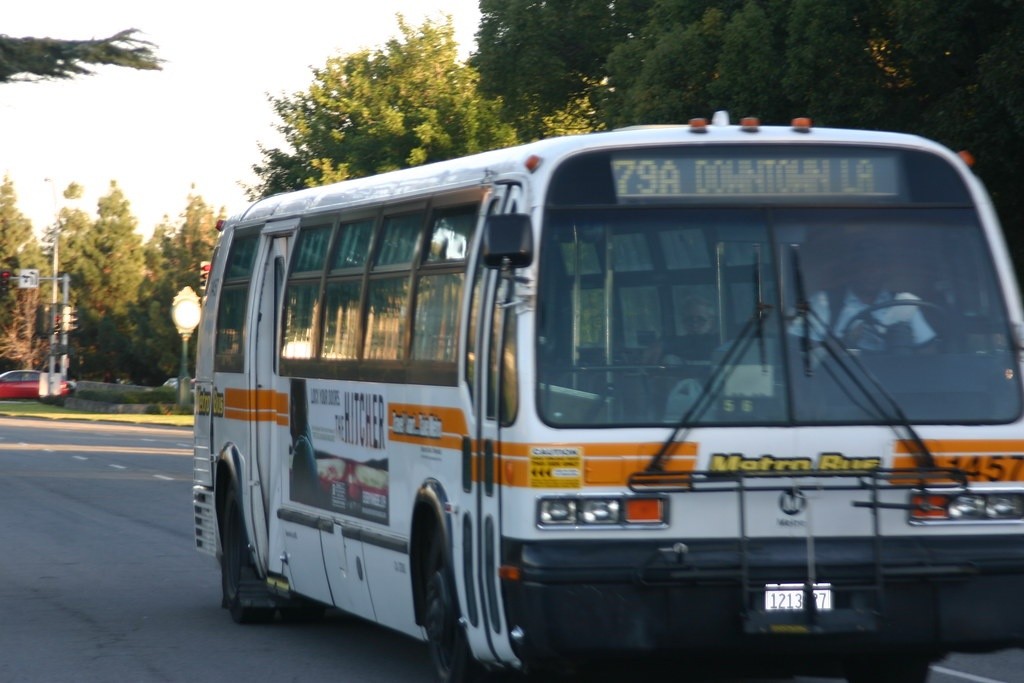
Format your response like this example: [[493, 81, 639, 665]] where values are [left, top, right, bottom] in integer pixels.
[[168, 286, 203, 414]]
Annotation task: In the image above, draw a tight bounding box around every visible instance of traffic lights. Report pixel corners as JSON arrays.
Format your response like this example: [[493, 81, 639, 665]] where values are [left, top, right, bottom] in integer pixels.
[[60, 304, 72, 331], [198, 260, 212, 289], [70, 307, 80, 331], [0, 270, 11, 293], [53, 314, 62, 333]]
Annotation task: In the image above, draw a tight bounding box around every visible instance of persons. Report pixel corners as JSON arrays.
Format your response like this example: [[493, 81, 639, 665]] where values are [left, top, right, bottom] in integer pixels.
[[784, 240, 939, 351], [637, 296, 721, 364]]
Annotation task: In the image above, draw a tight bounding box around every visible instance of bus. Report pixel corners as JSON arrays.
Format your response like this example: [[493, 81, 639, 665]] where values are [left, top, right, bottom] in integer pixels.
[[190, 108, 1024, 683]]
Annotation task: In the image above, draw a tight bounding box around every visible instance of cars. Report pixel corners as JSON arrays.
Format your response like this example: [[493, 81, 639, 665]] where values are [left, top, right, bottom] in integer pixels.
[[0, 369, 72, 401]]
